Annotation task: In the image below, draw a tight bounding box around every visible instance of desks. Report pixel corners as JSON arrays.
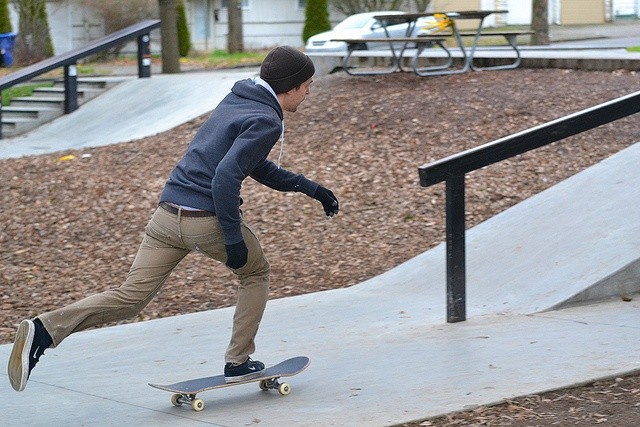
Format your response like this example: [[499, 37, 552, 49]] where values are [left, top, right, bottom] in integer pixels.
[[373, 10, 509, 75]]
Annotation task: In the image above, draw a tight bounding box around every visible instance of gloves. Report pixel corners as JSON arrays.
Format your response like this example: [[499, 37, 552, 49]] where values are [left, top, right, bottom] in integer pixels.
[[314, 185, 341, 217], [226, 240, 248, 269]]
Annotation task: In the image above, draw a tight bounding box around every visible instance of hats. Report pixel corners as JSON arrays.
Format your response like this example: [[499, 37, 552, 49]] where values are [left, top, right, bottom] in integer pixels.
[[260, 46, 314, 94]]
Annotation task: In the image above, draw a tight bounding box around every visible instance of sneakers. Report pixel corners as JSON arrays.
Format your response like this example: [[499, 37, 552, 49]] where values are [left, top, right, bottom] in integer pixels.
[[7, 319, 44, 391], [224, 358, 265, 383]]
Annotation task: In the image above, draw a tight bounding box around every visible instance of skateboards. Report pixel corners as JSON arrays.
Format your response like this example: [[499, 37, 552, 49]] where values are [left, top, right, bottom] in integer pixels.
[[148, 355, 312, 412]]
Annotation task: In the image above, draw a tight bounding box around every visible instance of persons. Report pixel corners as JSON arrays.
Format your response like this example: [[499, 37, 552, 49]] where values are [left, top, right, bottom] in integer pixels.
[[8, 46, 339, 392]]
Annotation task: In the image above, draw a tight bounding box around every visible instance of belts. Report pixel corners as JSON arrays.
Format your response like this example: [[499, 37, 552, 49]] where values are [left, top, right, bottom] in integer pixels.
[[160, 202, 215, 217]]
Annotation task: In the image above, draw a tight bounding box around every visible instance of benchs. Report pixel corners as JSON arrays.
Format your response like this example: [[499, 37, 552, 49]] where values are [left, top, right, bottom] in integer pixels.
[[330, 37, 446, 78], [417, 32, 536, 69]]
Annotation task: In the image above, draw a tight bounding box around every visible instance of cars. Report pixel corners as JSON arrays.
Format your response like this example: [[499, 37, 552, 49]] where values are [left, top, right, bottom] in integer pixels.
[[306, 10, 449, 51]]
